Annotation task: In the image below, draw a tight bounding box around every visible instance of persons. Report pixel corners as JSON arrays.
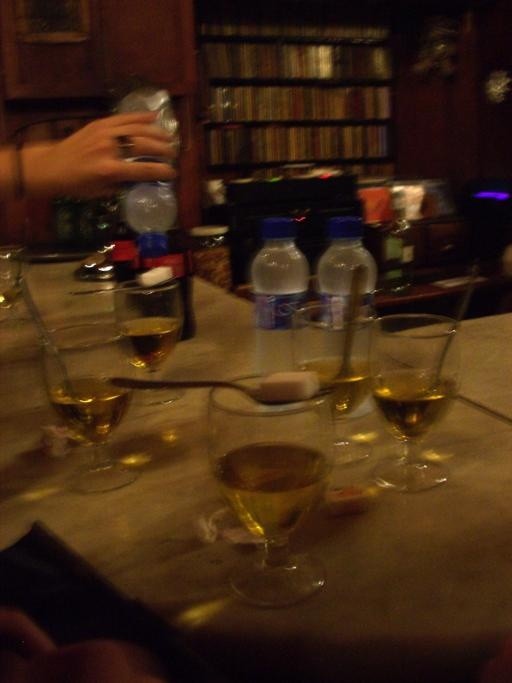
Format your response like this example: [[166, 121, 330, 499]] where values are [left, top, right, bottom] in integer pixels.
[[0, 110, 183, 204]]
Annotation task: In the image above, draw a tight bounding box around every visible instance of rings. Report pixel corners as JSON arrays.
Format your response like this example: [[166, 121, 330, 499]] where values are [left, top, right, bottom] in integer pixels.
[[114, 135, 135, 157]]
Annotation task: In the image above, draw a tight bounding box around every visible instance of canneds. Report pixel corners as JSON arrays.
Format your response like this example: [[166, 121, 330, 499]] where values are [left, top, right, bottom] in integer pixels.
[[189, 225, 235, 293]]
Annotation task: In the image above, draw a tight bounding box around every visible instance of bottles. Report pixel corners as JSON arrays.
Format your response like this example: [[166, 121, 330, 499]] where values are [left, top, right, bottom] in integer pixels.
[[119, 89, 180, 260], [252, 216, 379, 419]]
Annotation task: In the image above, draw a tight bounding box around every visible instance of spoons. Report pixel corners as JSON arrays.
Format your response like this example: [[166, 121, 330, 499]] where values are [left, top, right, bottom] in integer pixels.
[[29, 299, 93, 424], [103, 374, 339, 407]]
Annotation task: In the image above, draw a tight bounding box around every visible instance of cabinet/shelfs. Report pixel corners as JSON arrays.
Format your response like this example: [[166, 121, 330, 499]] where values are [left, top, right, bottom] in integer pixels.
[[197, 34, 394, 173]]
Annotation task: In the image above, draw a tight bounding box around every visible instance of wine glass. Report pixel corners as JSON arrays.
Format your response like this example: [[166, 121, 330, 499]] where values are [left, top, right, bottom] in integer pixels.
[[367, 313, 460, 494], [114, 281, 186, 405], [292, 299, 376, 467], [205, 375, 338, 607], [44, 324, 143, 495]]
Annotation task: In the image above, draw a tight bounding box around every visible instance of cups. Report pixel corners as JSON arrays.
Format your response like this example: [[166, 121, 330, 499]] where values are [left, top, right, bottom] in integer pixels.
[[1, 245, 24, 309]]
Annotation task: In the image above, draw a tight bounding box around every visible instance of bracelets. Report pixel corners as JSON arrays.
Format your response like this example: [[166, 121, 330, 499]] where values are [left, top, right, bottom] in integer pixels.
[[13, 145, 24, 198]]
